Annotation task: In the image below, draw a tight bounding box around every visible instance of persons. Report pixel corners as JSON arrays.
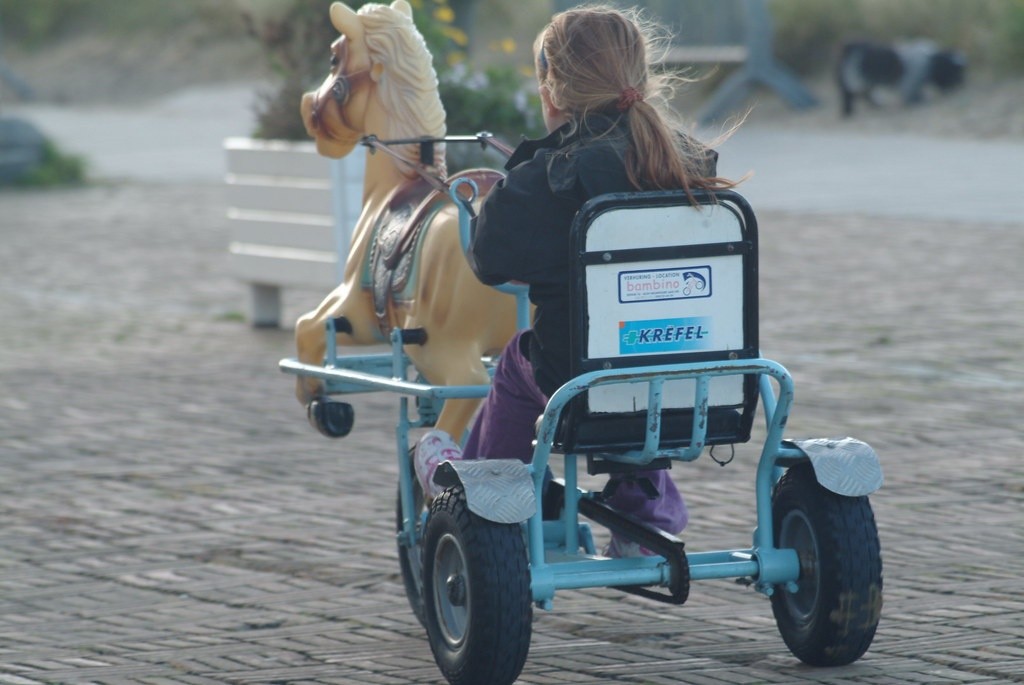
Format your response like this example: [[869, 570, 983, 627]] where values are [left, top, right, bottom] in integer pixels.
[[413, 6, 719, 558]]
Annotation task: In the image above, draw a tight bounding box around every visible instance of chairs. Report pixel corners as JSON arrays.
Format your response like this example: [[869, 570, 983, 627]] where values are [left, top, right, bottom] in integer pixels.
[[560, 189, 763, 442]]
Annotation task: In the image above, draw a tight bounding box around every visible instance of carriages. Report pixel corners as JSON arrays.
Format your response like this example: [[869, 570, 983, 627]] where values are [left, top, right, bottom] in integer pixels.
[[278, 0, 888, 685]]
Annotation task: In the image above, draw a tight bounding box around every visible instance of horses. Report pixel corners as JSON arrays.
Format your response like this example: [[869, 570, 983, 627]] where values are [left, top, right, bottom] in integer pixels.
[[293, 0, 544, 455]]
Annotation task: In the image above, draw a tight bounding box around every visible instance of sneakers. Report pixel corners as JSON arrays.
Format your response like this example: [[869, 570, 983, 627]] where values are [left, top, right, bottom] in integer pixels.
[[413, 430, 463, 499], [600, 533, 655, 559]]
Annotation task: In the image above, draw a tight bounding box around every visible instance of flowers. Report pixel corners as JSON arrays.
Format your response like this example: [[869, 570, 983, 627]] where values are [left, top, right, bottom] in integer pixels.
[[222, 0, 554, 151]]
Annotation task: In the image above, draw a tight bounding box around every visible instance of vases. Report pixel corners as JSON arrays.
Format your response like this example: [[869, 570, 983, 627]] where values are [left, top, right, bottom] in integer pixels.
[[221, 132, 371, 327]]
